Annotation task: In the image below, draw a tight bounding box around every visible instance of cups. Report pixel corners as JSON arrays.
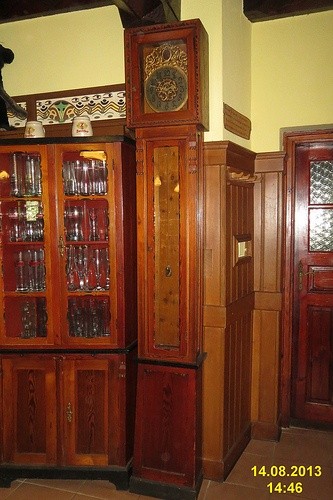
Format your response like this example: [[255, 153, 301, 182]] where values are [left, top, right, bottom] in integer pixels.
[[14, 249, 47, 291], [24, 120, 46, 139], [7, 201, 44, 242], [72, 116, 93, 137], [88, 208, 99, 242], [62, 159, 108, 196], [64, 205, 84, 242], [25, 155, 40, 196], [9, 153, 24, 197], [19, 302, 35, 338], [67, 296, 112, 338], [37, 300, 48, 337]]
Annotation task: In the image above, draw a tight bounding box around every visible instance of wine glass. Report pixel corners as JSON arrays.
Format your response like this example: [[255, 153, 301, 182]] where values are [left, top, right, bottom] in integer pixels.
[[91, 249, 105, 292], [64, 245, 76, 291], [106, 247, 111, 289], [73, 245, 88, 292]]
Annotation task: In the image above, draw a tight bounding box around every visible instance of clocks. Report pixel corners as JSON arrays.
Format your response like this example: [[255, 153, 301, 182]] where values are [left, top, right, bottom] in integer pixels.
[[123, 17, 210, 133]]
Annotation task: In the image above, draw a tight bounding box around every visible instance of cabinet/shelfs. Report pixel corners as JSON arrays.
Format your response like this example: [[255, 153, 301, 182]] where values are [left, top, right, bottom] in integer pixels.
[[0, 135, 136, 352], [0, 342, 137, 492]]
